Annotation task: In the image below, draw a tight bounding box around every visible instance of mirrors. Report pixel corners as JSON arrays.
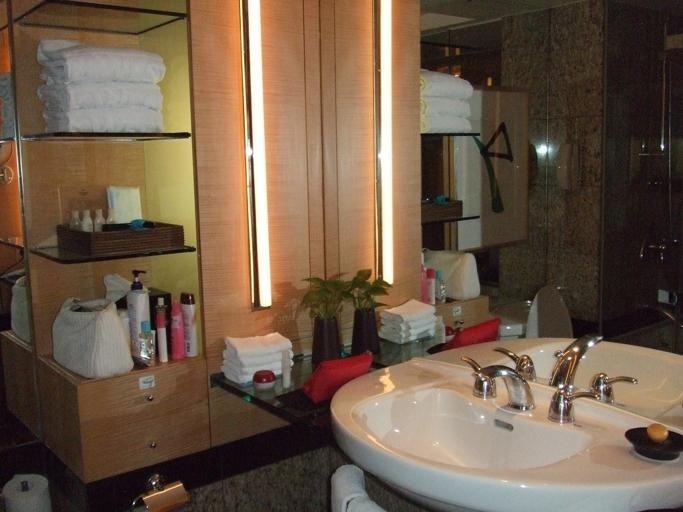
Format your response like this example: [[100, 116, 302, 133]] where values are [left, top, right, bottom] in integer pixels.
[[370, 2, 683, 361]]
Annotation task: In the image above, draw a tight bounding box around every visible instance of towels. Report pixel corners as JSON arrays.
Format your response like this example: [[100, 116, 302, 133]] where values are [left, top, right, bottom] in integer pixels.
[[37, 107, 165, 134], [34, 81, 163, 115], [377, 297, 437, 344], [419, 116, 472, 133], [419, 98, 471, 119], [419, 70, 473, 100], [34, 37, 167, 86], [345, 496, 386, 511], [221, 334, 292, 385], [329, 464, 368, 512]]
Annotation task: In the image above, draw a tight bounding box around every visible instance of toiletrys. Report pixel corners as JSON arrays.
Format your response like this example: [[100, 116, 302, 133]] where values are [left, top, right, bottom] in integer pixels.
[[253, 370, 276, 390], [282, 351, 290, 388], [139, 321, 155, 365], [445, 326, 455, 342], [69, 210, 80, 231], [93, 209, 106, 232], [436, 271, 446, 304], [427, 269, 436, 304], [80, 210, 94, 232], [106, 208, 117, 224], [156, 314, 168, 362], [434, 318, 445, 345]]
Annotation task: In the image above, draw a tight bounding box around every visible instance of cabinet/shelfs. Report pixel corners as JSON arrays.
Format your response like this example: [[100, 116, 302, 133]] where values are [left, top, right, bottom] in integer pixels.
[[423, 86, 527, 252], [4, 0, 211, 486], [419, 132, 491, 330]]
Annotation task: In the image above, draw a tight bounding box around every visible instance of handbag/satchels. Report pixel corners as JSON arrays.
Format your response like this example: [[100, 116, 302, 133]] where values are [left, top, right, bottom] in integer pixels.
[[53, 297, 136, 379]]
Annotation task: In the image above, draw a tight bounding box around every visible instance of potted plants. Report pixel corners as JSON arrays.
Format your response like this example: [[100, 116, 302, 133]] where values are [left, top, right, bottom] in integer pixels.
[[338, 268, 393, 356], [298, 271, 353, 367]]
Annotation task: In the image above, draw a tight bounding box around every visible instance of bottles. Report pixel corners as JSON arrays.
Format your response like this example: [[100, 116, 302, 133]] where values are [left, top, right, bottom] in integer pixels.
[[170, 291, 198, 362], [137, 320, 154, 366], [419, 251, 447, 307], [69, 208, 115, 233]]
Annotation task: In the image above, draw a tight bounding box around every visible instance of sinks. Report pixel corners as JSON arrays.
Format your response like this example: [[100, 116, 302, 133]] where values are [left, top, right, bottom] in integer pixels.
[[350, 382, 600, 512], [488, 338, 683, 424]]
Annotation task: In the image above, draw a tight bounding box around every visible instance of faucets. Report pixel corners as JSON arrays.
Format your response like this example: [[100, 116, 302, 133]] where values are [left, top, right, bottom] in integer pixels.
[[472, 364, 537, 413], [649, 238, 667, 262], [548, 333, 604, 390]]
